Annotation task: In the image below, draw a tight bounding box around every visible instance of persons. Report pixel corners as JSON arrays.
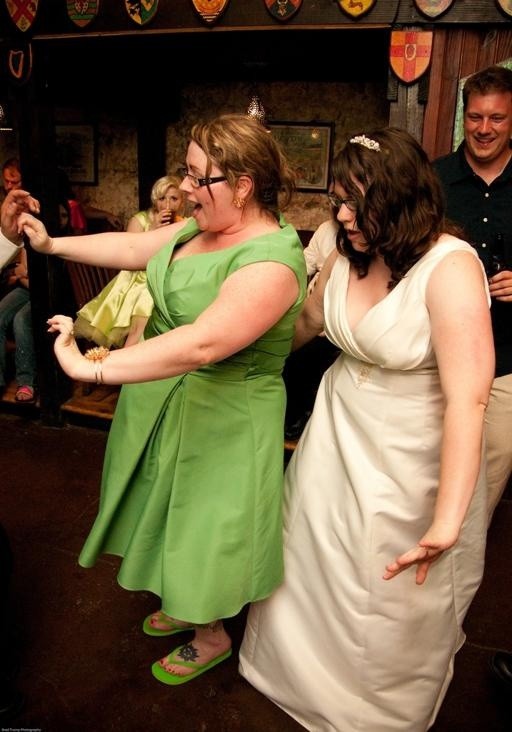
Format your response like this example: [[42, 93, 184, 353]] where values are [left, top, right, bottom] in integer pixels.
[[1, 156, 124, 403], [16, 112, 308, 684], [74, 175, 188, 350], [302, 216, 339, 277], [235, 126, 495, 730], [428, 63, 511, 532]]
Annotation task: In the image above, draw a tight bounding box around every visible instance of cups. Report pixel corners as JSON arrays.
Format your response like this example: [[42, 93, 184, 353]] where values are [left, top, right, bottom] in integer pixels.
[[160, 202, 176, 225], [485, 258, 503, 307]]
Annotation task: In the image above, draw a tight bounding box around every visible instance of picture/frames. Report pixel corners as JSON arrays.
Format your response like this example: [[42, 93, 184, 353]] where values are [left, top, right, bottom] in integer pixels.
[[261, 117, 336, 193], [51, 119, 100, 188]]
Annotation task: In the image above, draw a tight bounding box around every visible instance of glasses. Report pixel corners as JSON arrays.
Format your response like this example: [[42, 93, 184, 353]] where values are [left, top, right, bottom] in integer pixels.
[[182, 168, 226, 189], [327, 192, 358, 211]]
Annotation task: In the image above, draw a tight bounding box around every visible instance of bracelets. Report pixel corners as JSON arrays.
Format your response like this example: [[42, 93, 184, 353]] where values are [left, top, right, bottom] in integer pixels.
[[82, 345, 110, 387]]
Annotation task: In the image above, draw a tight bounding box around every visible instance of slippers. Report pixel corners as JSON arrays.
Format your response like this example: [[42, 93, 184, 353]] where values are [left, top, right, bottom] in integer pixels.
[[151, 645, 232, 686], [142, 611, 195, 637]]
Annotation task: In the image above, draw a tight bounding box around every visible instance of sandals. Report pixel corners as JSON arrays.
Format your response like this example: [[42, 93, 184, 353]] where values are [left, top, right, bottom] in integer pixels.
[[16, 384, 36, 404]]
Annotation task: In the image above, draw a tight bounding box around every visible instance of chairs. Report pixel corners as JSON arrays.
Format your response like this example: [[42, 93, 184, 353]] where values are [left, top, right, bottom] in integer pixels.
[[54, 226, 137, 402]]
